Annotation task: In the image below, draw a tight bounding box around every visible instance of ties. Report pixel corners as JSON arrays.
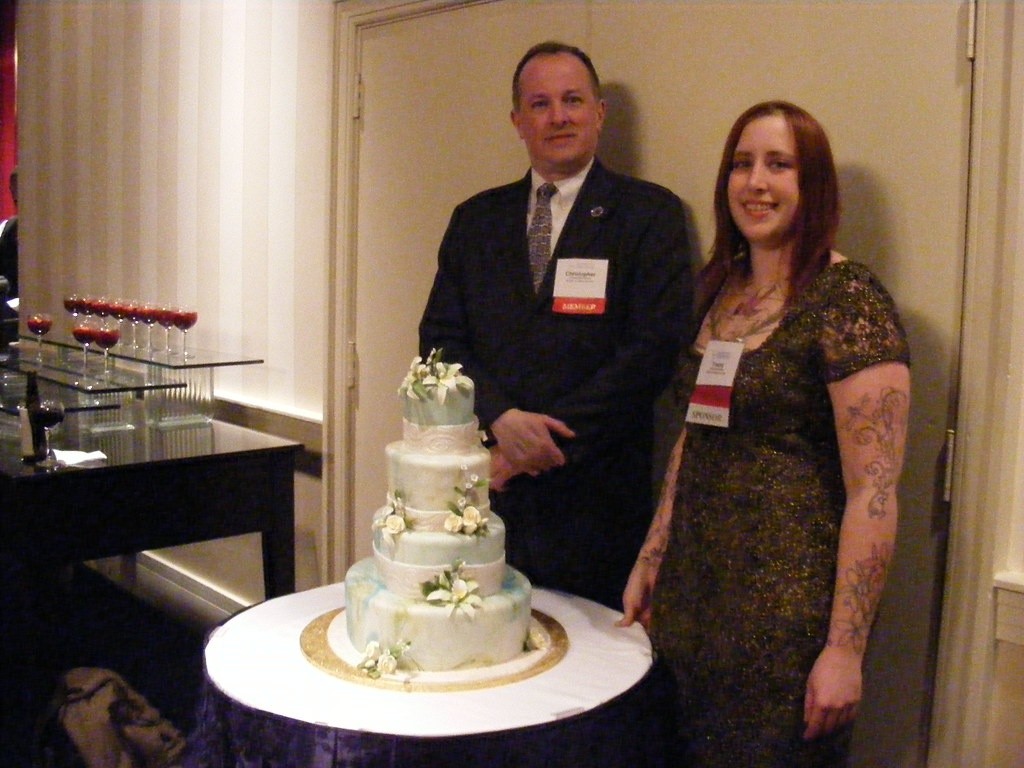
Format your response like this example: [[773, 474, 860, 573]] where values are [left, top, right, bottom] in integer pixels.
[[525, 182, 559, 295]]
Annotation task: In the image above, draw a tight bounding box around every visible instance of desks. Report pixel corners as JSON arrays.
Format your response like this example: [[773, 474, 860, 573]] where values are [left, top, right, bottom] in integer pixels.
[[176, 582, 695, 768], [0, 417, 306, 595]]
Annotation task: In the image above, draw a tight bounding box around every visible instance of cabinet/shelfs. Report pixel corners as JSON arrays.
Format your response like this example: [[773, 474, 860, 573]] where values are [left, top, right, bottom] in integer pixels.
[[0, 330, 264, 416]]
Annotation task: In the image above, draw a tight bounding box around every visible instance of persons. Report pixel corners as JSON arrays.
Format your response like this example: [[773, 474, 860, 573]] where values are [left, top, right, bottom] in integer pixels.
[[416, 39, 700, 614], [614, 100, 912, 768]]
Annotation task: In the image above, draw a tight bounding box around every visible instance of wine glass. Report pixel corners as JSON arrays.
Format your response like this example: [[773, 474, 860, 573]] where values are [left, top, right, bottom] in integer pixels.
[[63, 293, 198, 360], [73, 314, 100, 375], [93, 319, 121, 376], [36, 400, 66, 471], [26, 312, 54, 365]]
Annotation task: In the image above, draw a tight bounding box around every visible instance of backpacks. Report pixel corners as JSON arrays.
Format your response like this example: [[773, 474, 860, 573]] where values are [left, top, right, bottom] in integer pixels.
[[30, 666, 186, 768]]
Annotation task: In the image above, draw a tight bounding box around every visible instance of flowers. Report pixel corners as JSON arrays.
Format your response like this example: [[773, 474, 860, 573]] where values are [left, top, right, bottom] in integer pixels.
[[371, 487, 417, 552], [354, 638, 457, 679], [420, 558, 481, 620], [395, 347, 475, 407], [445, 462, 493, 544]]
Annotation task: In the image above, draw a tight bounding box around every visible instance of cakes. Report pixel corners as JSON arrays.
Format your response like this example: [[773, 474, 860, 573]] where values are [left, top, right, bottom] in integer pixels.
[[344, 345, 545, 679]]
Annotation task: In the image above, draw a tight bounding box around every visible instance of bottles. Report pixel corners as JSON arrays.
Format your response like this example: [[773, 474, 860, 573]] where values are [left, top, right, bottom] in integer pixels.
[[17, 370, 48, 464]]
